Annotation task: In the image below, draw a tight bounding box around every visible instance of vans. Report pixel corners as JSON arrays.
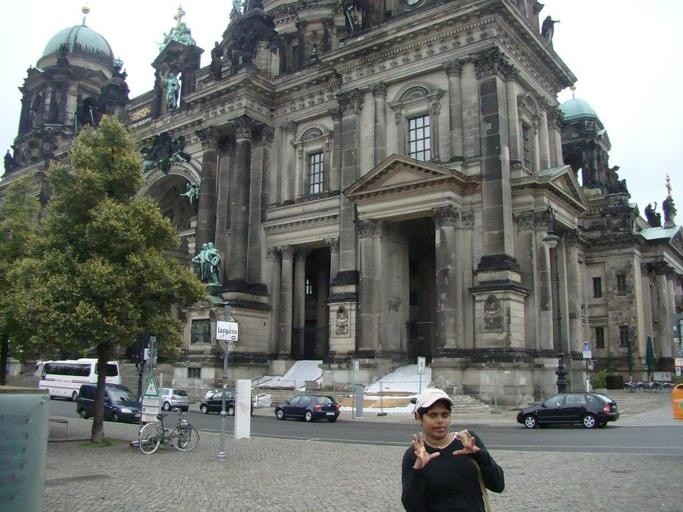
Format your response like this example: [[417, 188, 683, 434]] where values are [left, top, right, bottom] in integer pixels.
[[76, 382, 141, 425]]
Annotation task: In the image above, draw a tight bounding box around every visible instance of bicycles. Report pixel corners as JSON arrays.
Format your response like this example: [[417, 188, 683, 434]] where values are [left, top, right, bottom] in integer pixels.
[[136, 406, 200, 456]]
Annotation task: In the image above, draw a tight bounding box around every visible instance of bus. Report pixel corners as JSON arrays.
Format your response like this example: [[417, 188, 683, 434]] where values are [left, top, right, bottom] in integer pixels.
[[33, 357, 120, 402]]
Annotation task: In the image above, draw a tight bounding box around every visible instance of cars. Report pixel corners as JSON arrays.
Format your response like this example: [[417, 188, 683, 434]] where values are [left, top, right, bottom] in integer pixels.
[[197, 390, 254, 417], [135, 387, 191, 413], [272, 393, 340, 424], [516, 390, 620, 430]]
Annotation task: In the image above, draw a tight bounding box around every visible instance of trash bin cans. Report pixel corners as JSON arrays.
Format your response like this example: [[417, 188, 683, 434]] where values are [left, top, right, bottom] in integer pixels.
[[671, 383, 683, 420]]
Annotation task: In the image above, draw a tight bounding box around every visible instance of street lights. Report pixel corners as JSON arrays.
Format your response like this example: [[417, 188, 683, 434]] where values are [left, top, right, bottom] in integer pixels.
[[541, 199, 571, 394]]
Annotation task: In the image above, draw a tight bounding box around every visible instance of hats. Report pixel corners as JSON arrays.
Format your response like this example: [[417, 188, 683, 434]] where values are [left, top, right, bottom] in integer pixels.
[[415, 388, 453, 412]]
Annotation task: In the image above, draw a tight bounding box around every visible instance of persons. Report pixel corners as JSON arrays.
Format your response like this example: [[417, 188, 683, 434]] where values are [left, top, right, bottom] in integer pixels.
[[159, 71, 181, 110], [191, 242, 220, 283], [400, 387, 505, 512]]
